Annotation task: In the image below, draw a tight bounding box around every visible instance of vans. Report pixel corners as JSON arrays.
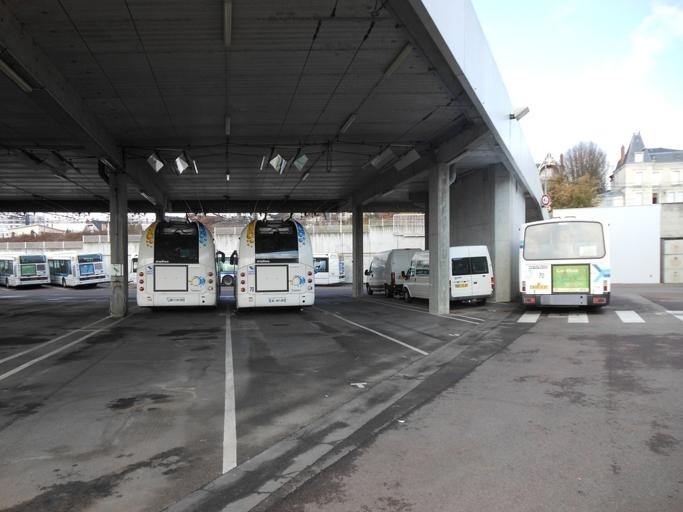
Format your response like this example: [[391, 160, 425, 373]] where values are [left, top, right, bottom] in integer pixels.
[[365, 245, 495, 306]]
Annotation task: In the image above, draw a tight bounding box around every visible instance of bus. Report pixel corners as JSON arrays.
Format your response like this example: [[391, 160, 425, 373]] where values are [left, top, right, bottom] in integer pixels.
[[132, 219, 345, 308], [0, 250, 107, 288], [520, 217, 611, 308]]
